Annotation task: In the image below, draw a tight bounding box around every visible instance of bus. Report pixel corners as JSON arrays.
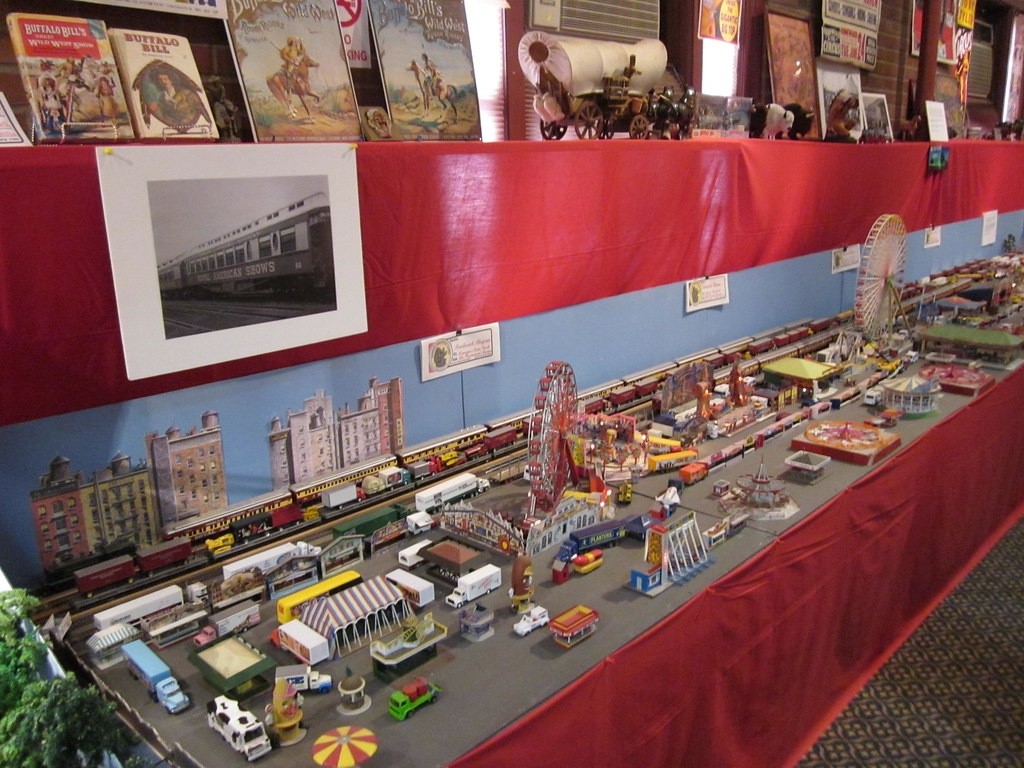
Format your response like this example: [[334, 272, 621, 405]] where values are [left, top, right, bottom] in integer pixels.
[[277, 570, 363, 625]]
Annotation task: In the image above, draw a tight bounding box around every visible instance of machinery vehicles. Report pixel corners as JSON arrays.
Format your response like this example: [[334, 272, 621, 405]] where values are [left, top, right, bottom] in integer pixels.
[[618, 480, 632, 505]]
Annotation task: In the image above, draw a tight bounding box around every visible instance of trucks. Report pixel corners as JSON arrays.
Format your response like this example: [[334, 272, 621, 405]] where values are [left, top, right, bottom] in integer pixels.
[[554, 519, 626, 565], [398, 539, 435, 571], [679, 463, 708, 486], [385, 568, 435, 609], [207, 694, 272, 761], [275, 664, 332, 693], [94, 473, 493, 631], [445, 564, 502, 609], [72, 272, 949, 603], [387, 676, 441, 722], [269, 618, 329, 666], [513, 606, 550, 637], [193, 599, 261, 647]]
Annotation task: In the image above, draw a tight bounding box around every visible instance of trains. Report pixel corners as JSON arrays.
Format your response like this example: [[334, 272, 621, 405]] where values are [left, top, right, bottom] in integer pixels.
[[156, 193, 335, 299]]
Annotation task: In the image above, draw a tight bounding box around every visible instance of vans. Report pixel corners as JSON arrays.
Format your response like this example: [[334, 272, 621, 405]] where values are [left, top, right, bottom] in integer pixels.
[[863, 385, 883, 406]]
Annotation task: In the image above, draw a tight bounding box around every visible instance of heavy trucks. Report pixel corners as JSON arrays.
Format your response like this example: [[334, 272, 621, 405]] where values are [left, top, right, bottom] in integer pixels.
[[121, 639, 190, 714]]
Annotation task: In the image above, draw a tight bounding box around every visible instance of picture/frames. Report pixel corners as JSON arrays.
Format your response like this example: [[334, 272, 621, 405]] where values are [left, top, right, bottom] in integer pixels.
[[762, 6, 822, 141], [857, 91, 895, 143], [816, 53, 866, 142]]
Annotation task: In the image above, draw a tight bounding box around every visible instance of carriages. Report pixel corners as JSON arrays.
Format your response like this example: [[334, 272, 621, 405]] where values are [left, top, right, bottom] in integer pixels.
[[517, 31, 696, 140]]
